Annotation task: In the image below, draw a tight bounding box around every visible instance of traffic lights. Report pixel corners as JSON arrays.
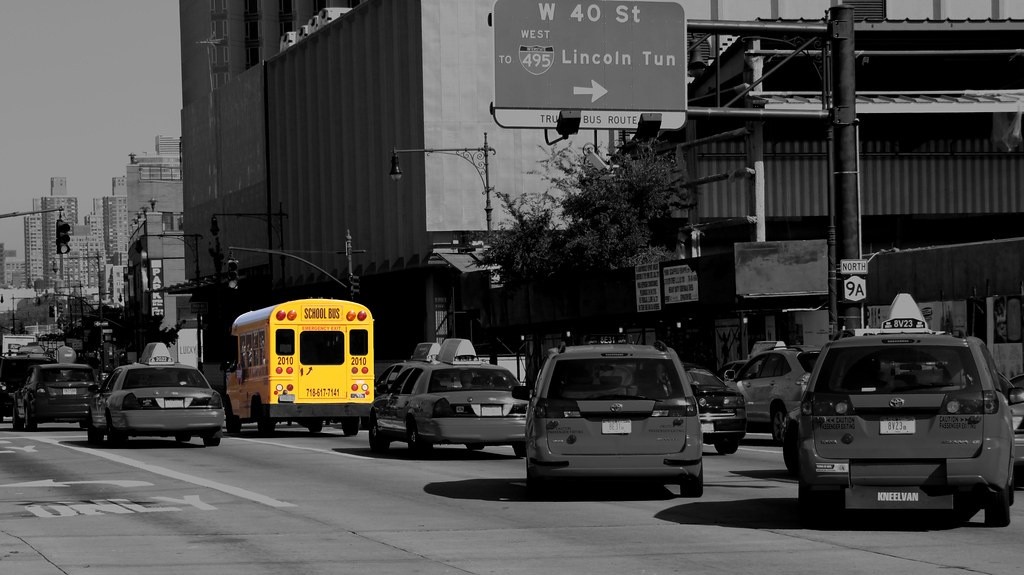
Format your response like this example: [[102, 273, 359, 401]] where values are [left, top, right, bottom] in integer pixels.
[[227, 260, 240, 290], [56, 219, 70, 254]]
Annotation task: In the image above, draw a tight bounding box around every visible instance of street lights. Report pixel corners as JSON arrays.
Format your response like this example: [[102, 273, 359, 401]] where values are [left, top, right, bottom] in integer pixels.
[[135, 233, 203, 288], [10, 294, 41, 332], [53, 251, 103, 321], [389, 132, 497, 364], [210, 202, 289, 283]]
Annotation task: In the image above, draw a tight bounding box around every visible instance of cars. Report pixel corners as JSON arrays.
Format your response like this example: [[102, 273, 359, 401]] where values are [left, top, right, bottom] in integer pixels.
[[374, 343, 441, 398], [369, 338, 529, 462], [680, 362, 748, 454], [87, 342, 224, 446], [783, 369, 1024, 474], [715, 340, 787, 381], [1009, 374, 1024, 388], [799, 293, 1015, 527]]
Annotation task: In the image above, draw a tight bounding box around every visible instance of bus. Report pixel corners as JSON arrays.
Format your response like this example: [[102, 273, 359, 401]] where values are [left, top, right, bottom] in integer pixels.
[[222, 298, 375, 437]]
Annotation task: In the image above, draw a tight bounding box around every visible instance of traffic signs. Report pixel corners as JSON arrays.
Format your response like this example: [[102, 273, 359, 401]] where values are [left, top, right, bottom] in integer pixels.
[[495, 0, 687, 130]]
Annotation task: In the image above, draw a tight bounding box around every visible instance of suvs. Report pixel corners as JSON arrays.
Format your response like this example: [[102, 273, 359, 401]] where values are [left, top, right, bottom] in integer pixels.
[[723, 346, 822, 447], [525, 341, 703, 498], [0, 353, 59, 422], [13, 363, 99, 432]]
